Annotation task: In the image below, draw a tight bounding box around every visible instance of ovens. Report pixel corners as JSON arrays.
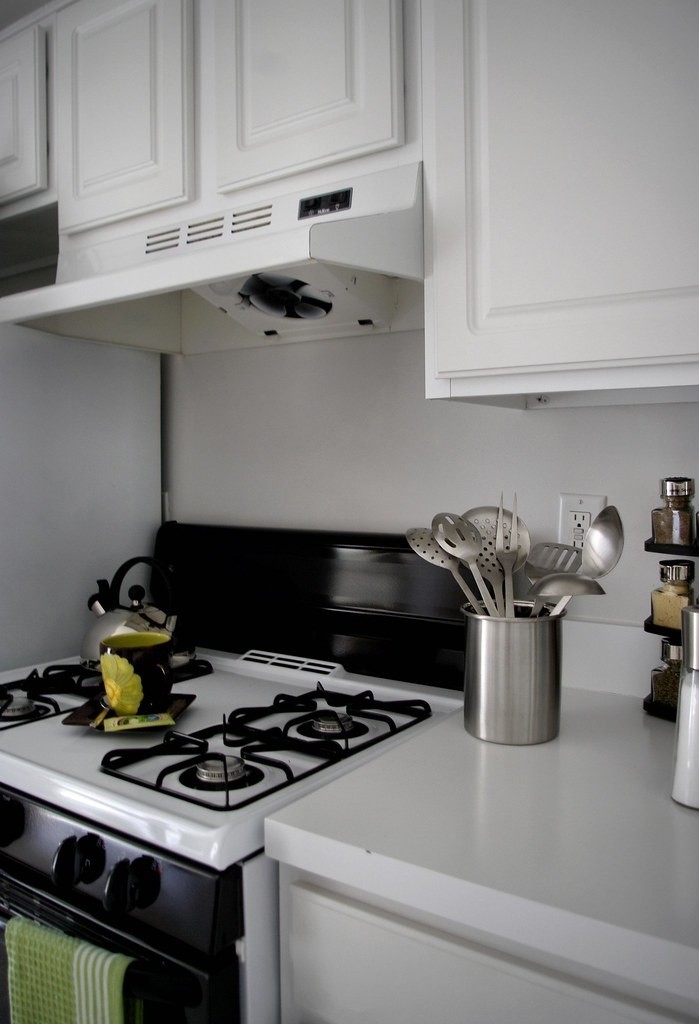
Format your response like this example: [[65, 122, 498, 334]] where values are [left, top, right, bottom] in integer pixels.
[[0, 786, 246, 1024]]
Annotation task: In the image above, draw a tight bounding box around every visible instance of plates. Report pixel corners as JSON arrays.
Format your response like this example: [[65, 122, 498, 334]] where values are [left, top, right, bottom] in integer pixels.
[[60, 689, 197, 733]]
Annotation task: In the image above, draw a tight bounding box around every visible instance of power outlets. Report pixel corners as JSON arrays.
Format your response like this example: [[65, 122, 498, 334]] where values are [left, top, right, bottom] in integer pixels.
[[557, 494, 607, 572]]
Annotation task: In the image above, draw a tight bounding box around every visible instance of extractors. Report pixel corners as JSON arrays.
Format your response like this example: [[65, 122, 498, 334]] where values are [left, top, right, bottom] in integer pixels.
[[0, 161, 422, 357]]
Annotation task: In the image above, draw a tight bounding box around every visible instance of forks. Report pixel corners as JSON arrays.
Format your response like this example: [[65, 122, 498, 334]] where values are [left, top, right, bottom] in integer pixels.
[[497, 491, 519, 617]]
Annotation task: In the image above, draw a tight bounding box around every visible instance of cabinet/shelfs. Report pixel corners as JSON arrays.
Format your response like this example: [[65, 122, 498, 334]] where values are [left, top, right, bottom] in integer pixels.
[[0, 0, 57, 221], [47, 0, 404, 254], [420, 0, 699, 410]]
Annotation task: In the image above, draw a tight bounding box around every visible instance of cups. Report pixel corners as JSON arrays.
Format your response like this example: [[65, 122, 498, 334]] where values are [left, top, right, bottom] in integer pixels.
[[100, 632, 173, 718], [460, 600, 567, 745]]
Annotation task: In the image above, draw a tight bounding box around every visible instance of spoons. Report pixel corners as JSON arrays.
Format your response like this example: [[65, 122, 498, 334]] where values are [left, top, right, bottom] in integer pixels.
[[528, 572, 608, 616], [548, 505, 625, 616], [89, 693, 111, 728]]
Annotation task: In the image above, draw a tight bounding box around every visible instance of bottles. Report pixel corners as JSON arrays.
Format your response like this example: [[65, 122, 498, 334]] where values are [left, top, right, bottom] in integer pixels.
[[650, 559, 695, 629], [669, 604, 699, 810], [650, 637, 683, 705], [651, 477, 697, 546]]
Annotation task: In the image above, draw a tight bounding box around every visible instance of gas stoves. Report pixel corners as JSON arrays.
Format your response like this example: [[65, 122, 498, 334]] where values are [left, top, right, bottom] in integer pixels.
[[0, 521, 502, 872]]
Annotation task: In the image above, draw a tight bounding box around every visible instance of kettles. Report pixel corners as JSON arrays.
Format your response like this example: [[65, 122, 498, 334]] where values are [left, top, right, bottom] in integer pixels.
[[77, 557, 178, 673]]
